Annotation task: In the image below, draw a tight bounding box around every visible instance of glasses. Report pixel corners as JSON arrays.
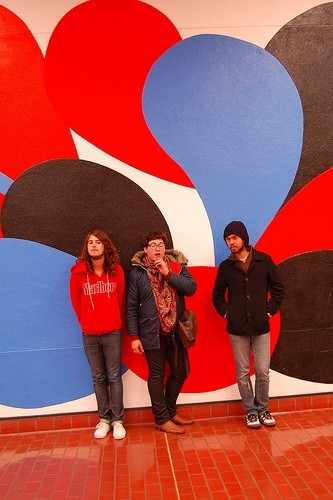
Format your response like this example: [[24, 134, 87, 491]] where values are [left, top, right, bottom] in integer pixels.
[[147, 241, 165, 250]]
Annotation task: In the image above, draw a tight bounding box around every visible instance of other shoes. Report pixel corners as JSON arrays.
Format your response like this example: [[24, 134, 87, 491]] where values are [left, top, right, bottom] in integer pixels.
[[112, 423, 127, 440], [94, 422, 111, 439], [172, 414, 194, 425], [155, 420, 185, 434]]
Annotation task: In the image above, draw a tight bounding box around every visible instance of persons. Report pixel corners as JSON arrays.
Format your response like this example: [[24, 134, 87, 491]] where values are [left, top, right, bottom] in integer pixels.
[[212, 221, 285, 429], [70, 229, 126, 439], [126, 230, 197, 434]]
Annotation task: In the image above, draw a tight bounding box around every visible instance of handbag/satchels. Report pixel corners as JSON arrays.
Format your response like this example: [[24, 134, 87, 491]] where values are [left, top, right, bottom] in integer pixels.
[[176, 309, 197, 349]]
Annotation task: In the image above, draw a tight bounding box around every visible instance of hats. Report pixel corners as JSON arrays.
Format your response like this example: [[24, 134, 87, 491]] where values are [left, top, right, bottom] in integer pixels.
[[223, 221, 249, 246]]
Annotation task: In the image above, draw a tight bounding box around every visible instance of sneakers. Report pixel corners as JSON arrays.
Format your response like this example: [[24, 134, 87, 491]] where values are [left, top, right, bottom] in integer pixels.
[[259, 411, 276, 426], [246, 413, 260, 428]]
